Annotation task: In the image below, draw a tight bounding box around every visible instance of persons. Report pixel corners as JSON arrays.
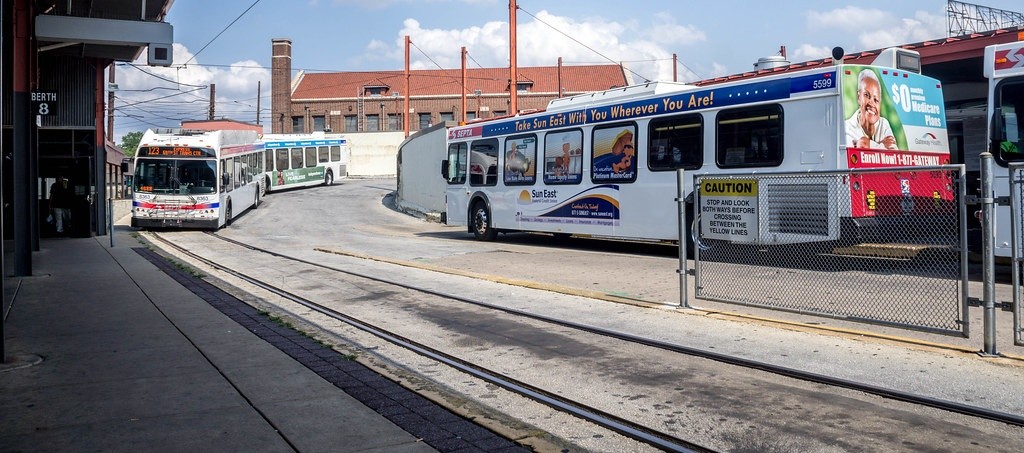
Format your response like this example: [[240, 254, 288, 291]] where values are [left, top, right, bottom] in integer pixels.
[[49, 174, 63, 232], [845, 68, 899, 150]]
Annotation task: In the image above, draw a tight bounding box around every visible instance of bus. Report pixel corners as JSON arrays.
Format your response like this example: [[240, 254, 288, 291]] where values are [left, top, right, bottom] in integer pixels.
[[263, 133, 350, 194], [981, 41, 1024, 281], [434, 45, 956, 262], [119, 129, 267, 232]]
